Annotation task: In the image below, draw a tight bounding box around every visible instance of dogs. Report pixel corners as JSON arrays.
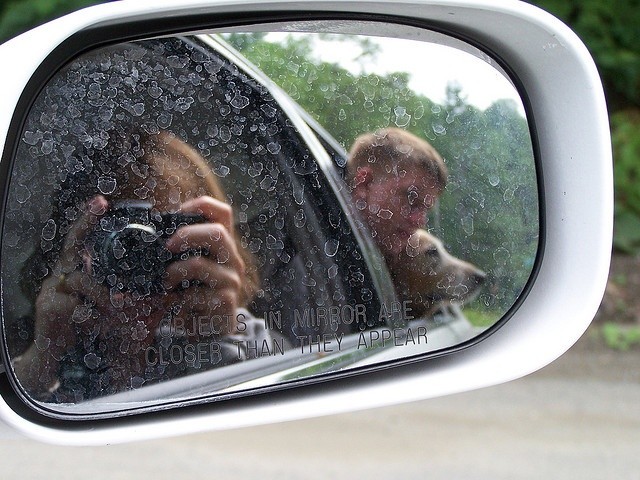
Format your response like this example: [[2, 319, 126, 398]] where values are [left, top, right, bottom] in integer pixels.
[[385, 229, 485, 322]]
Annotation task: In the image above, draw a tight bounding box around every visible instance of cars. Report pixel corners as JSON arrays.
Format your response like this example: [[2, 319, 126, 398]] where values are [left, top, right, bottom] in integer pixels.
[[0, 31, 477, 414]]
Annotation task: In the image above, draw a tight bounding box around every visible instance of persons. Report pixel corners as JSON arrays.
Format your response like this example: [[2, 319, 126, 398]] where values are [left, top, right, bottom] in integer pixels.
[[345, 129, 450, 257], [11, 130, 274, 405]]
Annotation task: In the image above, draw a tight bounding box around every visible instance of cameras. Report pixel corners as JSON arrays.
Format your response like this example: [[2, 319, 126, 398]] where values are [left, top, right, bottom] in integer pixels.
[[89, 199, 211, 299]]
